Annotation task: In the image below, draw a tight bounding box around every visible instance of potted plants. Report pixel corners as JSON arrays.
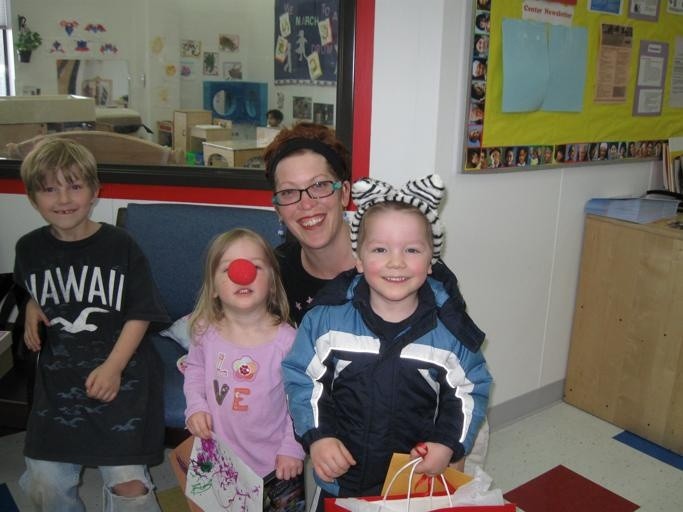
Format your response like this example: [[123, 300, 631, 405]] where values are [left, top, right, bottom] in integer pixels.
[[16, 32, 43, 63]]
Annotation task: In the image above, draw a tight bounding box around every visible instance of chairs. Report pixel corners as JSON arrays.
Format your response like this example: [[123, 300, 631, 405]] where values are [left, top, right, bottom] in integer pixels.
[[7, 131, 186, 164], [14, 203, 303, 450]]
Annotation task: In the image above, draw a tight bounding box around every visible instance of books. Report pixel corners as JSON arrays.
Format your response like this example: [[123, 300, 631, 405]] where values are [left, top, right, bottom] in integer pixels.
[[261, 458, 306, 511]]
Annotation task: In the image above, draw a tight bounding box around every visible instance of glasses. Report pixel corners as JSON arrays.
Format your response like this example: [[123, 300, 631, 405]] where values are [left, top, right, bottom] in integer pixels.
[[272, 180, 342, 206]]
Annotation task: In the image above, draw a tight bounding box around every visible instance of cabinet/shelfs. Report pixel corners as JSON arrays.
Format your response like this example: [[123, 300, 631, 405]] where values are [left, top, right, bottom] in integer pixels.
[[563, 212, 681, 457], [190, 117, 233, 148], [199, 125, 280, 168], [171, 106, 212, 153]]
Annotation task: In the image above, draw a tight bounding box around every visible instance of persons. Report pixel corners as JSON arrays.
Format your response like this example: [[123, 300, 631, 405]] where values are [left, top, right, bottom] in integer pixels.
[[259, 121, 468, 474], [278, 173, 494, 512], [178, 224, 307, 511], [7, 137, 172, 512], [467, 0, 490, 169], [261, 111, 284, 128], [487, 139, 662, 169]]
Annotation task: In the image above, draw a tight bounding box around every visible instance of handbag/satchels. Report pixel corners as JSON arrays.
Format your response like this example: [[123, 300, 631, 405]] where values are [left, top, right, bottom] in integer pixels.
[[324, 457, 516, 512]]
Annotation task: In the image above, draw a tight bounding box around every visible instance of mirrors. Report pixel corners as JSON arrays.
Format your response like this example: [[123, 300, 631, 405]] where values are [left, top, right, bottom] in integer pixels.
[[0, 0, 352, 194], [55, 57, 129, 109]]
